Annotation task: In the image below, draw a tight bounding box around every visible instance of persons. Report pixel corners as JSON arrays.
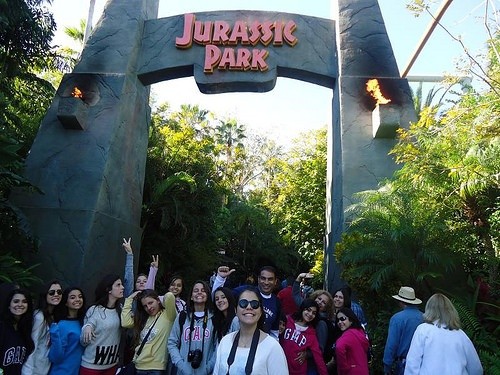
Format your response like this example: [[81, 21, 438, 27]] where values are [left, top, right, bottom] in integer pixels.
[[403, 293, 483, 375], [0, 237, 370, 375], [383, 286, 425, 375]]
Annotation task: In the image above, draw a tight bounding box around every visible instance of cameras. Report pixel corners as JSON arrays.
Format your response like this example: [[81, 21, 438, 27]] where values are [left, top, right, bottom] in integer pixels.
[[188, 349, 203, 369]]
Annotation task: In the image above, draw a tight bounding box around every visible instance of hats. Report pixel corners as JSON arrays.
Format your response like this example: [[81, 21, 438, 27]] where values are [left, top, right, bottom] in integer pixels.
[[393, 287, 422, 305]]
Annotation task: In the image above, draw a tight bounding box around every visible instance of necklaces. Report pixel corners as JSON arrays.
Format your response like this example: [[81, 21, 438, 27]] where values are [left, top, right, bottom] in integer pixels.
[[240, 337, 252, 347]]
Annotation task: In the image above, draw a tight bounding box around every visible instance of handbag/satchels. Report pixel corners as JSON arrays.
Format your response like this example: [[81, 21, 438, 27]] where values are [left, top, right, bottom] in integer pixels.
[[117, 364, 136, 375], [365, 332, 373, 367]]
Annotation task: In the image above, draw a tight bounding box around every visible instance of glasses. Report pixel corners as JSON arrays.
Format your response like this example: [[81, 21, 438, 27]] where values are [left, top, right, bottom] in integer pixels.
[[237, 299, 261, 309], [49, 288, 61, 296], [336, 316, 349, 322]]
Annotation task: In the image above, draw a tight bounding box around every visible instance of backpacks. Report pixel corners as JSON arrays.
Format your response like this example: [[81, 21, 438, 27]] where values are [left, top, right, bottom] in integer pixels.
[[318, 316, 337, 362]]
